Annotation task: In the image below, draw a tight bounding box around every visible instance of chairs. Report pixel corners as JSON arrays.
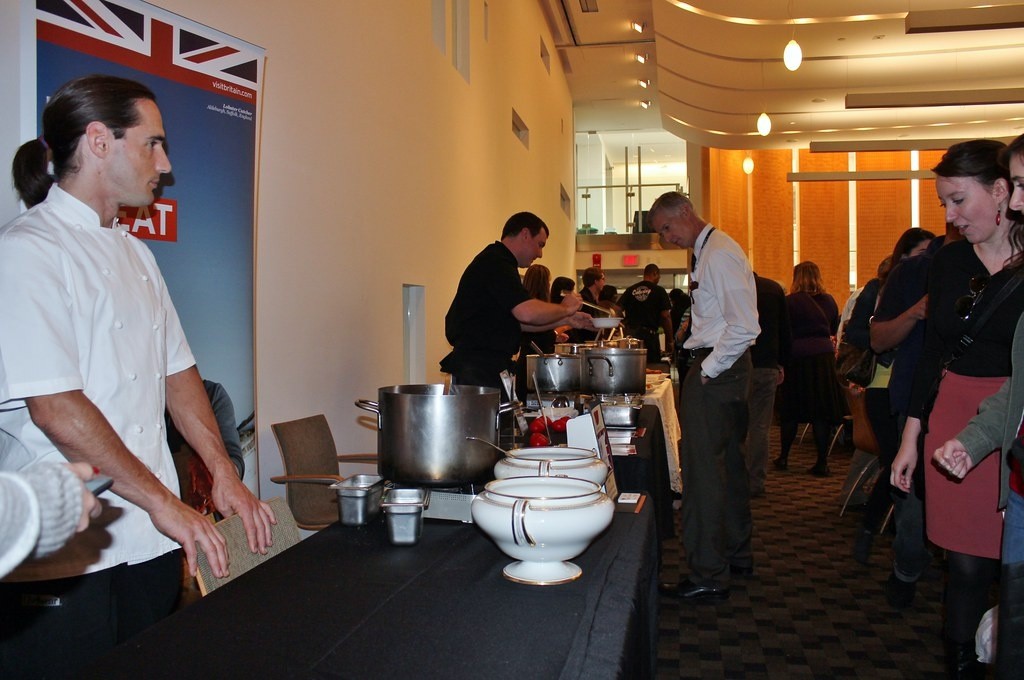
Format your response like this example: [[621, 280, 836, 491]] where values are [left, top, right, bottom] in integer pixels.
[[798, 419, 848, 458], [268, 414, 378, 531], [839, 382, 898, 535], [193, 495, 302, 599]]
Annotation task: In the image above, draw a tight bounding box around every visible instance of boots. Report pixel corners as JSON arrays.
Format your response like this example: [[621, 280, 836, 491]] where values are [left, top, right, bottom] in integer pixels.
[[941, 636, 977, 680]]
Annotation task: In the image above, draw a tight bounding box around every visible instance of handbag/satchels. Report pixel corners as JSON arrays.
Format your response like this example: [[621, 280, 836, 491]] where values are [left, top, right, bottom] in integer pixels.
[[877, 344, 900, 368], [913, 397, 933, 501], [836, 342, 877, 388]]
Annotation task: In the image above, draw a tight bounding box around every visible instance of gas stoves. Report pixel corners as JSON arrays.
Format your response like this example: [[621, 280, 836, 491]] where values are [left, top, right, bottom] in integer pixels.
[[524, 395, 641, 414], [382, 480, 492, 524]]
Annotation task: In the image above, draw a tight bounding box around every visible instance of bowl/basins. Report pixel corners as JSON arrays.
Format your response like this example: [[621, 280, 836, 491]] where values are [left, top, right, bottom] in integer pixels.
[[589, 317, 623, 328], [472, 476, 615, 586], [494, 449, 608, 488]]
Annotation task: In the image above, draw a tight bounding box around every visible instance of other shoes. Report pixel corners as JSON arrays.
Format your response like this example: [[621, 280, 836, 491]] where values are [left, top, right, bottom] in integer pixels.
[[853, 526, 873, 564], [809, 465, 831, 478], [773, 457, 788, 469], [888, 571, 916, 607]]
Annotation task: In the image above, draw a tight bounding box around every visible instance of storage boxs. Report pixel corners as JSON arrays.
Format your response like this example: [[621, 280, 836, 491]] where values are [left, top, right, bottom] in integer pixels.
[[378, 487, 430, 544], [328, 473, 385, 528], [583, 395, 641, 426]]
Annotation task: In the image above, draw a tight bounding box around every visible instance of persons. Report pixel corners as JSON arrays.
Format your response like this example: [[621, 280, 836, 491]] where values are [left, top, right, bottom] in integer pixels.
[[846, 227, 937, 569], [646, 192, 762, 601], [516, 264, 793, 497], [0, 462, 102, 579], [837, 255, 893, 351], [869, 222, 967, 608], [439, 212, 599, 429], [164, 380, 246, 523], [0, 74, 277, 578], [886, 140, 1024, 680], [759, 261, 849, 477], [933, 133, 1024, 680]]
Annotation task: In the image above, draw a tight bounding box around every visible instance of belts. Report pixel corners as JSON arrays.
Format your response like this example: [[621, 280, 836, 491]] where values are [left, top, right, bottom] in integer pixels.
[[690, 347, 713, 360]]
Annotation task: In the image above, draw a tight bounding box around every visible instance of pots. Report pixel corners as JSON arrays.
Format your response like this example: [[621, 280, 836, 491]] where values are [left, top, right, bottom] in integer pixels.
[[525, 336, 647, 394], [354, 383, 524, 484]]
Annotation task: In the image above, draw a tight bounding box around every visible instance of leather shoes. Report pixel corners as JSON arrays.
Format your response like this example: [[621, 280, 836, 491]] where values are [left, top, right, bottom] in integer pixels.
[[657, 578, 729, 602], [728, 563, 753, 576]]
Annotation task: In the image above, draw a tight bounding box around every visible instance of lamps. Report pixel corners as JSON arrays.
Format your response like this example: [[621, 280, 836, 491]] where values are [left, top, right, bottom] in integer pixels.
[[640, 100, 652, 109], [638, 78, 651, 90], [634, 50, 650, 64], [631, 17, 648, 34], [756, 59, 772, 137], [743, 113, 754, 175], [782, 0, 803, 72]]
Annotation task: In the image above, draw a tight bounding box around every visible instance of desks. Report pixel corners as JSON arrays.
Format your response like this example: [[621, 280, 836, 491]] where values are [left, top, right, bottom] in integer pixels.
[[501, 365, 676, 540], [58, 491, 664, 680]]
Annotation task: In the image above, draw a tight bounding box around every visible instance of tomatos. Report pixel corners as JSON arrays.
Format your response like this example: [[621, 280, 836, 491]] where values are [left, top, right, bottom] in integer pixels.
[[527, 414, 571, 446]]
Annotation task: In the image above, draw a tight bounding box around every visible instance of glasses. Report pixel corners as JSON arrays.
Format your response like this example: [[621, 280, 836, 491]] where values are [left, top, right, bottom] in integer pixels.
[[953, 273, 989, 321]]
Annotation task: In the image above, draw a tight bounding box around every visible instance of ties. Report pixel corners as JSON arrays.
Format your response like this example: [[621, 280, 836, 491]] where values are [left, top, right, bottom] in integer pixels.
[[680, 227, 716, 348]]
